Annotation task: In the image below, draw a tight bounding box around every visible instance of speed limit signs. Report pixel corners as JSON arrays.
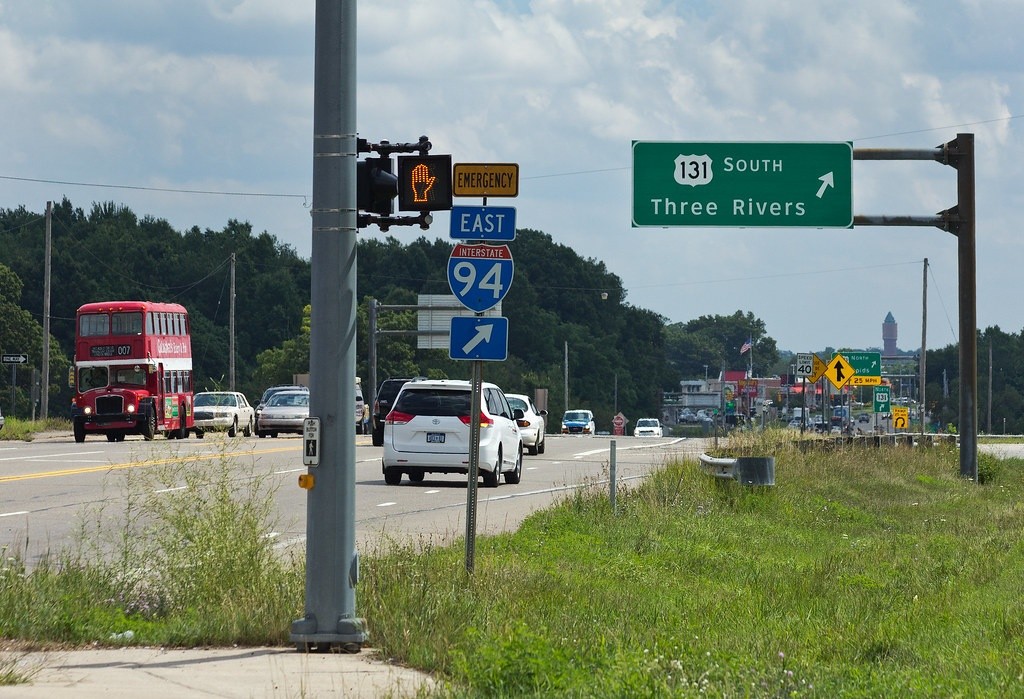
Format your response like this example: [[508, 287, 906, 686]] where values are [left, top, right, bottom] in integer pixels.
[[796, 352, 813, 376]]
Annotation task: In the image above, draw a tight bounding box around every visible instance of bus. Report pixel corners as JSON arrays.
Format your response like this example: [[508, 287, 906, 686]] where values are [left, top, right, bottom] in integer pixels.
[[70, 300, 194, 442]]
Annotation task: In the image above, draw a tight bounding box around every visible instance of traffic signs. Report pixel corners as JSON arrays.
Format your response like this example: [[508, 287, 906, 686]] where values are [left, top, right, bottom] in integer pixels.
[[1, 353, 29, 364], [831, 352, 882, 386], [630, 139, 856, 230]]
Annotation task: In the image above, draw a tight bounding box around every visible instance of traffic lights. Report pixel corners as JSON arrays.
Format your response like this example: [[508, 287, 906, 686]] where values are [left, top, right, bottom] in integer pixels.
[[398, 155, 454, 213]]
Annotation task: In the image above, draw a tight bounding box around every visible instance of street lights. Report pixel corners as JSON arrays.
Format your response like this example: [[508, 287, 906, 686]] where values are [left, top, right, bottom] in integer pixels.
[[702, 365, 708, 382]]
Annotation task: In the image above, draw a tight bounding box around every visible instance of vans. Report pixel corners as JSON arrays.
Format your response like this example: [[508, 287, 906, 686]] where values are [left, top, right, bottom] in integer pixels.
[[559, 410, 597, 435]]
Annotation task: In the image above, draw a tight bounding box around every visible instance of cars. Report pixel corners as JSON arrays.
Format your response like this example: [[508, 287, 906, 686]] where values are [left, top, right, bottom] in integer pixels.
[[504, 393, 549, 456], [790, 395, 920, 437], [678, 407, 717, 426], [189, 392, 255, 438], [632, 418, 664, 438], [257, 391, 312, 439]]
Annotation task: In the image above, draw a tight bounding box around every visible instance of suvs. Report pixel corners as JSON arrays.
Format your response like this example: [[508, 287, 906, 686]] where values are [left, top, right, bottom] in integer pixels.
[[253, 385, 309, 436], [369, 376, 421, 447], [381, 375, 526, 487]]
